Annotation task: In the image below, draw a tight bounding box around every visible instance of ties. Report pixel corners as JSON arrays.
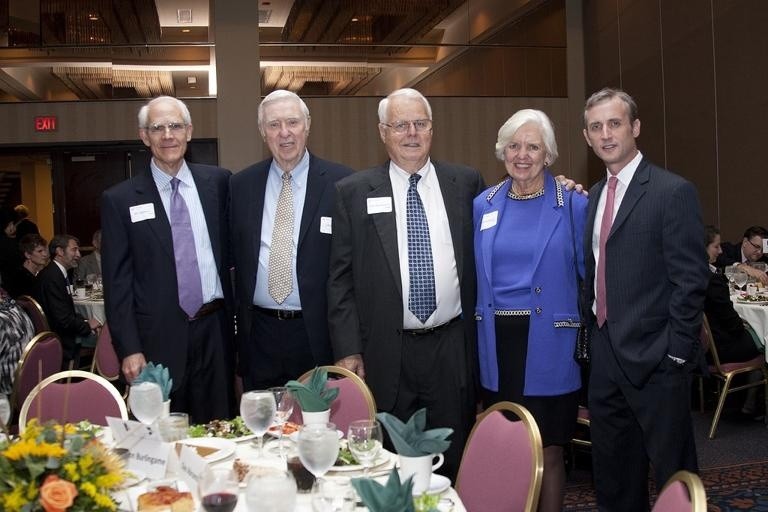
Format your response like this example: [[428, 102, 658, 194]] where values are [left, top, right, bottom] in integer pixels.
[[595, 176, 619, 329], [406, 173, 437, 325], [267, 171, 295, 305], [170, 176, 204, 320]]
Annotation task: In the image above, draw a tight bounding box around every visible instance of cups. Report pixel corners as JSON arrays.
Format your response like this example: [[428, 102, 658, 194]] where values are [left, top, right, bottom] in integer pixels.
[[301, 408, 331, 429], [75, 288, 85, 296], [749, 287, 757, 295], [160, 399, 171, 422], [105, 448, 130, 474], [159, 413, 189, 443], [398, 452, 444, 490], [245, 466, 298, 511]]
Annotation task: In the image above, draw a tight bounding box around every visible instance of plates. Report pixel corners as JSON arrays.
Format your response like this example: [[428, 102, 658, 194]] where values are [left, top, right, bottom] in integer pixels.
[[169, 438, 237, 462], [188, 421, 257, 442], [216, 465, 281, 486], [265, 423, 302, 436], [327, 447, 390, 472], [91, 299, 104, 301], [372, 473, 451, 495], [736, 301, 768, 306], [107, 471, 147, 490], [73, 297, 89, 301], [289, 429, 345, 441]]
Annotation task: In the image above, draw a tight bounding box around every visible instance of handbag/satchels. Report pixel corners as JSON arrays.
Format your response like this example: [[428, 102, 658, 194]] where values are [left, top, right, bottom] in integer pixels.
[[573, 325, 592, 365]]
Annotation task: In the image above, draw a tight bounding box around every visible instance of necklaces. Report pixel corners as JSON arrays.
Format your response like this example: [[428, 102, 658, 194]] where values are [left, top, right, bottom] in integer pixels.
[[510, 182, 544, 194]]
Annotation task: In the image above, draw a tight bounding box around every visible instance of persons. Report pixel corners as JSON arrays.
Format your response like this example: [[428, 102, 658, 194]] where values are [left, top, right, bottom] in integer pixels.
[[468, 107, 587, 510], [98, 94, 234, 427], [712, 226, 768, 287], [0, 202, 105, 432], [228, 88, 354, 393], [327, 87, 591, 487], [706, 223, 768, 424], [577, 86, 706, 510]]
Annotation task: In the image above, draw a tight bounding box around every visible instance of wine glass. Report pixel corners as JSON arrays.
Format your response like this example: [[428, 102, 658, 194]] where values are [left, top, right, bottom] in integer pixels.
[[240, 389, 278, 446], [312, 475, 358, 512], [349, 419, 383, 465], [128, 382, 166, 429], [74, 273, 103, 295], [265, 385, 296, 442], [296, 427, 342, 478], [723, 262, 768, 296]]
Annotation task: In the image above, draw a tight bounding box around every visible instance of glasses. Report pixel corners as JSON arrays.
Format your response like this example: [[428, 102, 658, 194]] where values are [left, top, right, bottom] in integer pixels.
[[144, 122, 188, 132], [381, 119, 434, 135], [747, 239, 762, 253]]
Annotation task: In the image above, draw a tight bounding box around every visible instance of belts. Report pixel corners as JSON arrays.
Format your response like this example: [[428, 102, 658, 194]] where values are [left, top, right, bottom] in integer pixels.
[[403, 314, 462, 336], [250, 304, 304, 321]]
